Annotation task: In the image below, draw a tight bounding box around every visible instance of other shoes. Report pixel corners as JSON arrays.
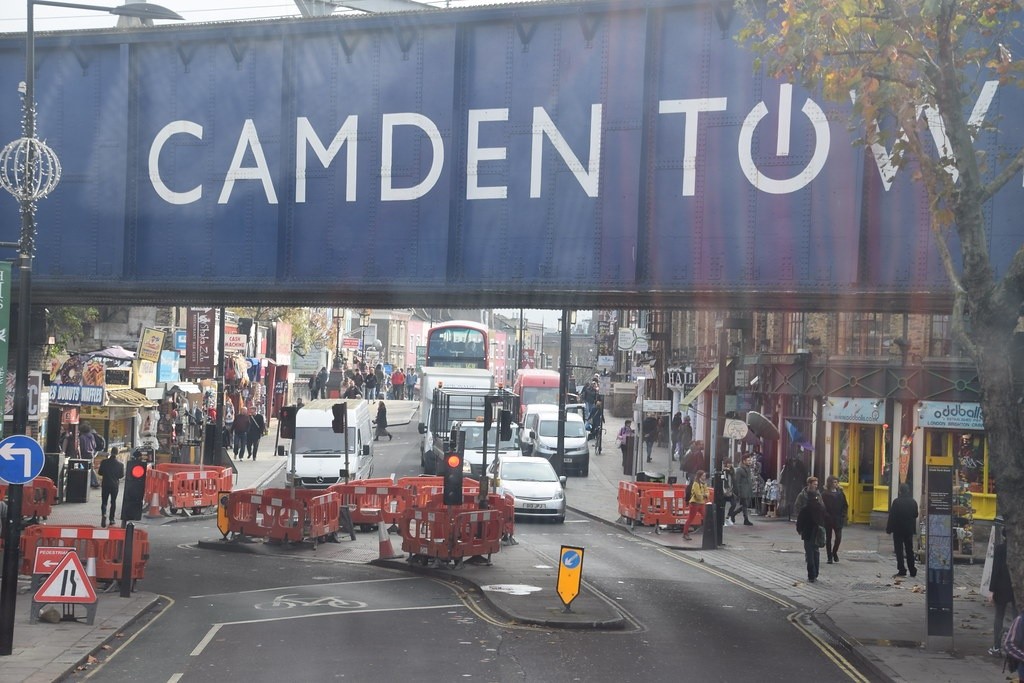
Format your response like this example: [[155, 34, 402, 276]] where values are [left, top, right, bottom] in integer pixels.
[[390, 434, 393, 440], [647, 456, 652, 463], [110, 519, 116, 525], [911, 567, 917, 577], [234, 452, 257, 462], [808, 577, 814, 582], [832, 552, 839, 562], [684, 534, 693, 540], [828, 560, 833, 564], [896, 570, 907, 576], [726, 517, 733, 526], [744, 519, 753, 525], [101, 515, 106, 528], [730, 513, 735, 523], [374, 438, 378, 441]]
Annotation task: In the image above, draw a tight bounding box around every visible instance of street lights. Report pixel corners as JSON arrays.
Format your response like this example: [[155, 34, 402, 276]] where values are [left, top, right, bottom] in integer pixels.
[[0, 0, 188, 649], [358, 308, 371, 372], [333, 306, 344, 357]]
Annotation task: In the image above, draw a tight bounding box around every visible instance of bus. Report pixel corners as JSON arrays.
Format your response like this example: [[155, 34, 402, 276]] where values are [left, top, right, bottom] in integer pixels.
[[511, 368, 561, 436], [425, 320, 492, 369]]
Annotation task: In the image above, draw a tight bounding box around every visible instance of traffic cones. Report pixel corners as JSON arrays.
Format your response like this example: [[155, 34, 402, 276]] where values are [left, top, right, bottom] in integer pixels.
[[143, 484, 165, 519], [373, 509, 405, 560], [85, 544, 99, 595]]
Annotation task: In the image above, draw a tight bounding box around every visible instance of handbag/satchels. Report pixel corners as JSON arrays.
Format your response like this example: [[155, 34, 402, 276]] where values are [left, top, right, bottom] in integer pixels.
[[615, 438, 621, 448], [814, 526, 826, 548], [585, 418, 594, 431], [261, 430, 266, 436]]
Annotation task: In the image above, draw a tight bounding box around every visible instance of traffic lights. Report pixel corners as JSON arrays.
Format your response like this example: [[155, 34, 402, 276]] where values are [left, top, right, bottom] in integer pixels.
[[120, 460, 146, 520], [280, 406, 296, 439], [443, 451, 464, 505], [332, 404, 344, 434], [500, 410, 513, 442]]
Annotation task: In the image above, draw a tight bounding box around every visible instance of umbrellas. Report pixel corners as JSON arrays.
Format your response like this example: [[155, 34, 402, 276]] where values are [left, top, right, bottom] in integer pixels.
[[740, 410, 815, 451]]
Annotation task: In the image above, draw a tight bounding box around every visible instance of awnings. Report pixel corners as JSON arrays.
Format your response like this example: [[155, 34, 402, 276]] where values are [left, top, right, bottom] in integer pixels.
[[107, 389, 150, 402]]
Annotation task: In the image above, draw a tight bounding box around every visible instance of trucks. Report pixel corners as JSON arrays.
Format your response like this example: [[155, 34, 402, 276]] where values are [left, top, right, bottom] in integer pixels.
[[418, 366, 496, 475], [431, 381, 525, 482]]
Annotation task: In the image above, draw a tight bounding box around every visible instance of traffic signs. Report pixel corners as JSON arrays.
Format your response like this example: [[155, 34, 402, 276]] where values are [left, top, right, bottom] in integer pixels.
[[33, 546, 76, 575]]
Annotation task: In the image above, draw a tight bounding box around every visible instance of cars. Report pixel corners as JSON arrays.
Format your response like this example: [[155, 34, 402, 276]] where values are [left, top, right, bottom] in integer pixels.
[[486, 456, 568, 524]]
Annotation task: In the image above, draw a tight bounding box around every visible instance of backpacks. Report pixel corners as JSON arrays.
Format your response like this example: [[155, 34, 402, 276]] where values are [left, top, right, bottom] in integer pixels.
[[679, 451, 701, 472], [92, 431, 105, 451], [686, 481, 701, 502]]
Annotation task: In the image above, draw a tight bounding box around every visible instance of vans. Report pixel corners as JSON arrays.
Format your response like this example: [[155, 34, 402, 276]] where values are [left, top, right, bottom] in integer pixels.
[[529, 412, 593, 477], [517, 404, 587, 458], [284, 398, 375, 488]]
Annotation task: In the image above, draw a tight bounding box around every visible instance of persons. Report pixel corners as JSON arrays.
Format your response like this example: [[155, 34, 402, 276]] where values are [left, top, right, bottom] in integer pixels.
[[309, 371, 320, 401], [246, 407, 265, 461], [297, 398, 305, 410], [820, 476, 848, 564], [886, 483, 918, 577], [462, 341, 483, 357], [681, 470, 710, 540], [78, 420, 100, 488], [671, 412, 692, 461], [317, 367, 328, 399], [643, 412, 657, 463], [230, 407, 251, 461], [617, 420, 634, 466], [794, 476, 831, 584], [98, 447, 125, 528], [721, 454, 753, 526], [342, 363, 384, 404], [62, 428, 82, 469], [386, 367, 418, 401], [683, 439, 707, 505], [373, 401, 392, 441], [579, 373, 605, 438]]
